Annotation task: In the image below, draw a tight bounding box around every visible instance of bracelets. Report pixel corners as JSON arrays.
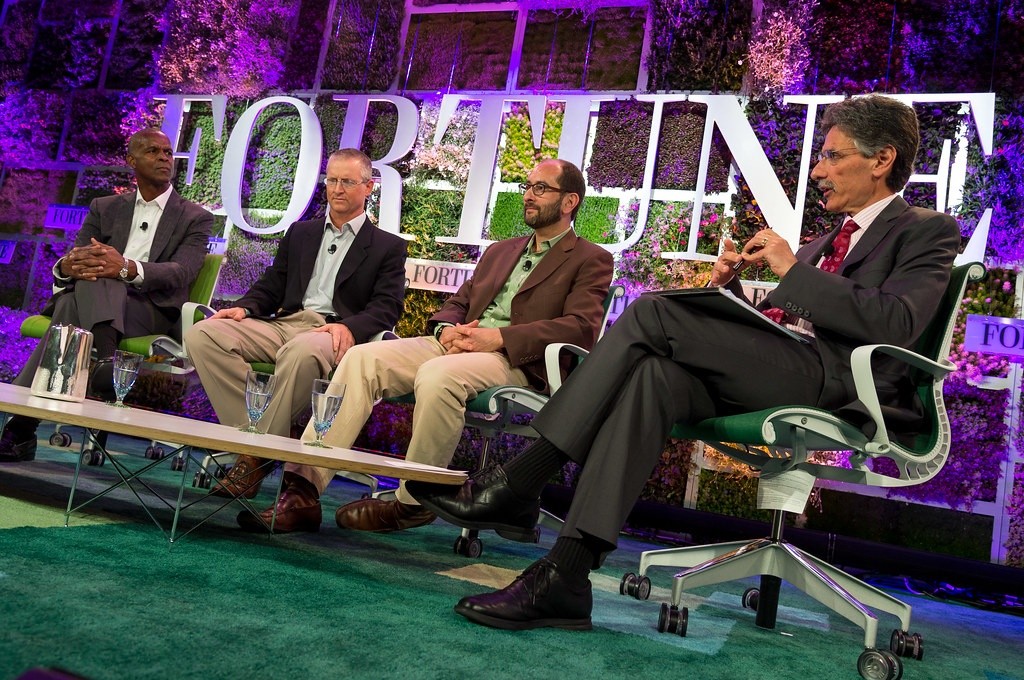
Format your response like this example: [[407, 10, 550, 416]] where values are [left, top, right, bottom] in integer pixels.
[[436, 325, 444, 339]]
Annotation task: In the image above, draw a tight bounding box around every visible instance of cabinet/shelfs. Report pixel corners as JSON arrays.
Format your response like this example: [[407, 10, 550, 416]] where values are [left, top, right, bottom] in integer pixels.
[[0, 0, 1023, 569]]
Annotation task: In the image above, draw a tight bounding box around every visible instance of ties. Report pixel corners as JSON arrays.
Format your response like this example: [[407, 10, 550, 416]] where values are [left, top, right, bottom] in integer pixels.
[[762, 221, 865, 323]]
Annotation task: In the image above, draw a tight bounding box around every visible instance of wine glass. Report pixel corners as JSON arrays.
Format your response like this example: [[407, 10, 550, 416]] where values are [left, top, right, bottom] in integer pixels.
[[105, 348, 144, 406], [300, 378, 346, 449], [236, 371, 276, 435]]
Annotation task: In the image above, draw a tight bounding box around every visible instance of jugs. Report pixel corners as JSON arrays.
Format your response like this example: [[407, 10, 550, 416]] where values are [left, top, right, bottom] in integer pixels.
[[29, 323, 93, 404]]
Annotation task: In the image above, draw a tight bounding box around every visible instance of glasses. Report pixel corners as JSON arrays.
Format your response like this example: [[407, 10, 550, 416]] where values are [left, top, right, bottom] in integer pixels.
[[324, 178, 367, 187], [817, 147, 857, 164], [516, 182, 561, 196]]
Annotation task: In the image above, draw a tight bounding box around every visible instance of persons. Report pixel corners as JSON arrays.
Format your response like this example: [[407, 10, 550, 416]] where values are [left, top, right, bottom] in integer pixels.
[[418, 91, 962, 629], [237, 154, 615, 534], [0, 127, 214, 464], [184, 148, 408, 497]]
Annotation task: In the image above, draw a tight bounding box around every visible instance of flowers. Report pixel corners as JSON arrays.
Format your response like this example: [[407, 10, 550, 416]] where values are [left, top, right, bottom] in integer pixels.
[[0, 0, 1024, 563]]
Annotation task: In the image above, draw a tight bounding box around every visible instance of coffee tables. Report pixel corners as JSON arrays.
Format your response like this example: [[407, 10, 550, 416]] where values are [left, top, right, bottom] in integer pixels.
[[0, 383, 468, 543]]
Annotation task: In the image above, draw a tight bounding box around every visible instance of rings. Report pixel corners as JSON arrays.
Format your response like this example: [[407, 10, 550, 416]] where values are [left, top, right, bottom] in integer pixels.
[[762, 239, 768, 245]]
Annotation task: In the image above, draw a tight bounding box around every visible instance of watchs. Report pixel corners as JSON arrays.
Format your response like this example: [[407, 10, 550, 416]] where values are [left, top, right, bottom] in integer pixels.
[[117, 257, 129, 281]]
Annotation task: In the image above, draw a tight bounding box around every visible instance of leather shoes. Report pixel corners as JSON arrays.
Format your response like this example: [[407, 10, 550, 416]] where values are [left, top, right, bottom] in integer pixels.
[[237, 483, 322, 533], [2, 423, 39, 463], [209, 455, 266, 498], [401, 462, 543, 544], [454, 556, 595, 633], [89, 351, 120, 400], [335, 498, 437, 532]]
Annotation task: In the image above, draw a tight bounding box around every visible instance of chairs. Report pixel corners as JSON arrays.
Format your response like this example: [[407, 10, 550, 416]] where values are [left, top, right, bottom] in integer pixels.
[[21, 253, 229, 468], [620, 262, 991, 680], [361, 283, 622, 559], [190, 360, 379, 490]]
